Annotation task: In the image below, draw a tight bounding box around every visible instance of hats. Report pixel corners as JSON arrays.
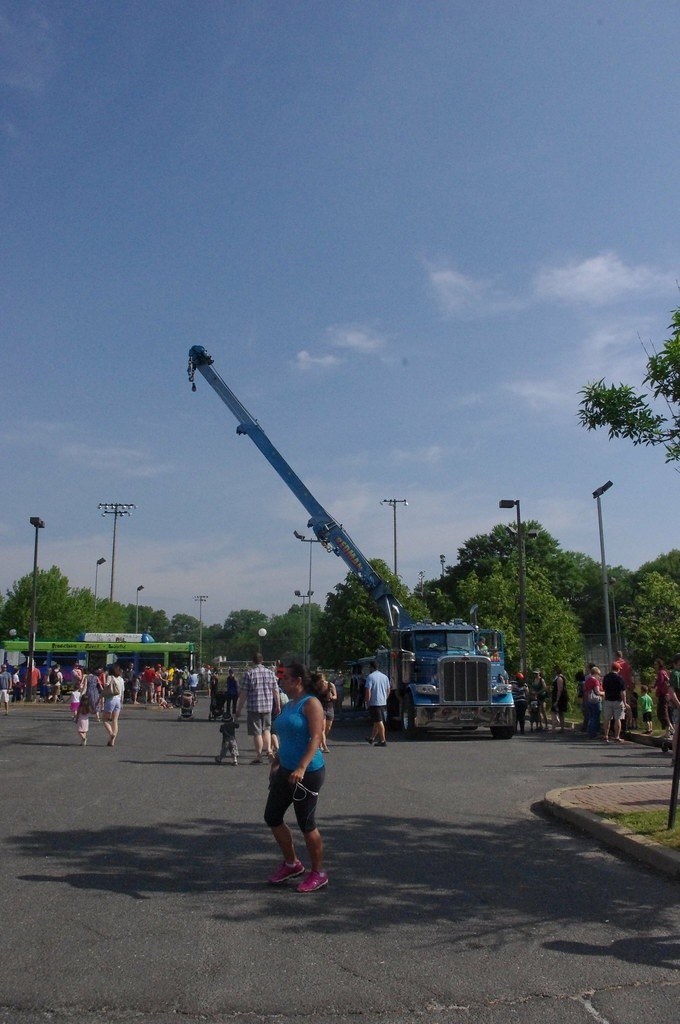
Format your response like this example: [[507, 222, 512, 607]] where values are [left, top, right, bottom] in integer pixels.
[[516, 673, 524, 680], [221, 712, 233, 723], [612, 662, 623, 670], [532, 668, 540, 673]]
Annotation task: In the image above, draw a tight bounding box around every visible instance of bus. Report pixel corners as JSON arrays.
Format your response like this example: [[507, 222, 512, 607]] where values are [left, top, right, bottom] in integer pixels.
[[0, 631, 207, 695]]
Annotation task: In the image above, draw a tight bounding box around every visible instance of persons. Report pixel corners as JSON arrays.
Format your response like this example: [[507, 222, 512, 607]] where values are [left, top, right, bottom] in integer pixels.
[[365, 660, 390, 747], [66, 664, 125, 747], [264, 663, 329, 892], [551, 665, 569, 734], [308, 673, 337, 753], [575, 651, 638, 743], [158, 696, 167, 709], [655, 653, 680, 766], [332, 675, 344, 714], [226, 669, 238, 720], [12, 660, 219, 705], [0, 664, 11, 716], [237, 653, 281, 763], [510, 667, 548, 734], [638, 685, 653, 735], [215, 713, 240, 766], [477, 636, 488, 653], [350, 675, 365, 711]]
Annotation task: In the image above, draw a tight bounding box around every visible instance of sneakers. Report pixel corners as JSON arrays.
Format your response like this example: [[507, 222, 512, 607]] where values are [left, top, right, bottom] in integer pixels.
[[268, 860, 304, 883], [296, 868, 329, 892]]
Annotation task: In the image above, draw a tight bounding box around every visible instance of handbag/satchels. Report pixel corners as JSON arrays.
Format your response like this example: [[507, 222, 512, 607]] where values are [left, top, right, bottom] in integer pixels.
[[587, 689, 601, 704], [552, 712, 560, 726], [97, 677, 104, 694], [101, 676, 119, 697]]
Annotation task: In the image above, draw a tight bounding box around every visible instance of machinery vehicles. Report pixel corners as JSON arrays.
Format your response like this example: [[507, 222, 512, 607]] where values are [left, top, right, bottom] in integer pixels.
[[186, 344, 518, 741]]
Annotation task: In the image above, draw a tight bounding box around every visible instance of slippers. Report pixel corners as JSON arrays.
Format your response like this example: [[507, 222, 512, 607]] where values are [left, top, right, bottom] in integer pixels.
[[268, 752, 275, 764]]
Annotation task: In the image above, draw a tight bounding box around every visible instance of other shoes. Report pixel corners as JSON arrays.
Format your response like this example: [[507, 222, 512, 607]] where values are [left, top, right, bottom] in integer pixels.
[[642, 730, 653, 735], [80, 738, 86, 745], [589, 735, 601, 739], [365, 735, 373, 744], [374, 741, 385, 746], [614, 738, 624, 743], [107, 742, 113, 747], [602, 736, 609, 742], [215, 756, 222, 764], [320, 749, 330, 753], [252, 759, 263, 765], [232, 763, 237, 766]]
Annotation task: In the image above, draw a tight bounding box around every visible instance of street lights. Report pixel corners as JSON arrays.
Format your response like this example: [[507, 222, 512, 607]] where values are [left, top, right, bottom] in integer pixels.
[[380, 499, 409, 574], [97, 503, 138, 605], [94, 557, 107, 614], [193, 595, 209, 668], [499, 499, 526, 673], [24, 516, 45, 702], [293, 530, 329, 670], [136, 585, 145, 633], [294, 589, 314, 666], [258, 627, 267, 656], [592, 479, 614, 673]]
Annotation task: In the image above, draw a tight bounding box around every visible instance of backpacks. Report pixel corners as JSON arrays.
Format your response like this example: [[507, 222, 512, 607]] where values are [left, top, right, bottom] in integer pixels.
[[50, 670, 59, 684]]
